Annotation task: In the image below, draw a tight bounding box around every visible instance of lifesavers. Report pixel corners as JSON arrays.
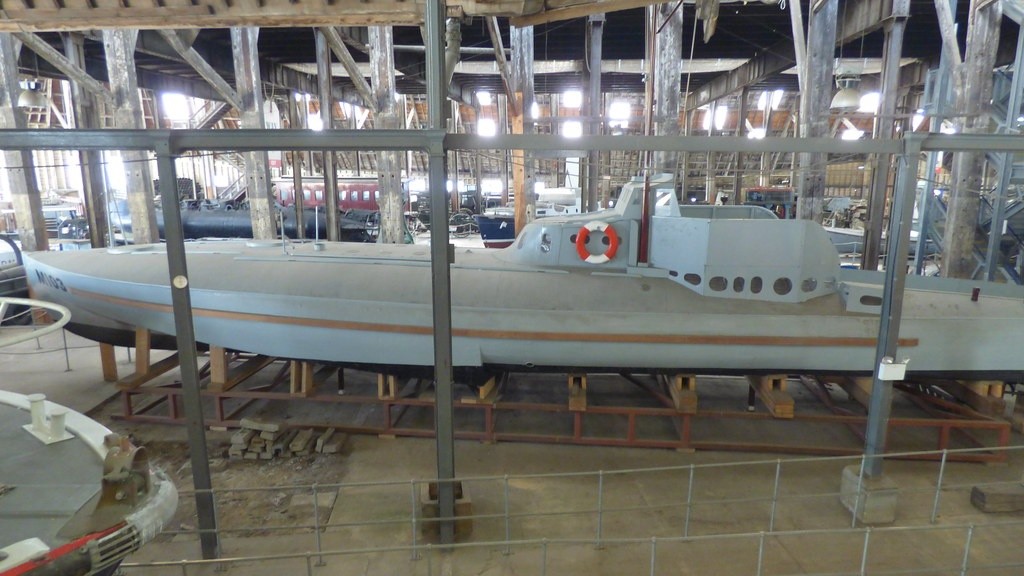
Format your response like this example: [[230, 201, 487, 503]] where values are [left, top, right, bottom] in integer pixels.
[[575, 220, 619, 264]]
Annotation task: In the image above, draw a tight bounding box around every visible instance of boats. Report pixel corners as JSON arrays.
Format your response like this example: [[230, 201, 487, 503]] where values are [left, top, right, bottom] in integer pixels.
[[0, 153, 1024, 576]]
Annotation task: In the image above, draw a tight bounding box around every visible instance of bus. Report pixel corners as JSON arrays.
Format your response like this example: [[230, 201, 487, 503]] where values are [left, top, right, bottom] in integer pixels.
[[226, 173, 411, 231]]
